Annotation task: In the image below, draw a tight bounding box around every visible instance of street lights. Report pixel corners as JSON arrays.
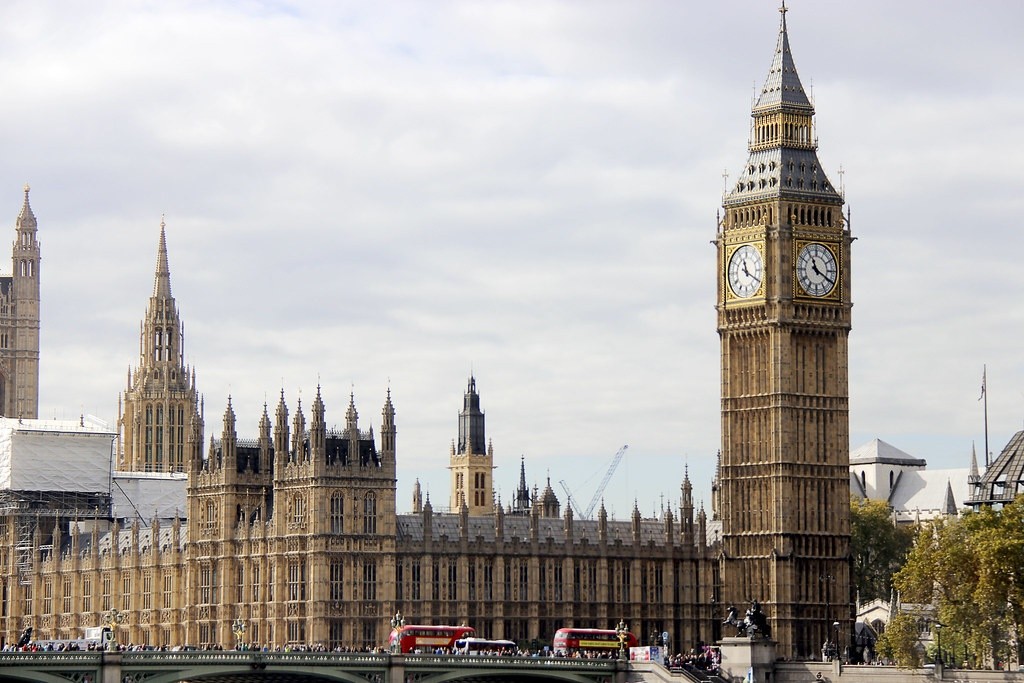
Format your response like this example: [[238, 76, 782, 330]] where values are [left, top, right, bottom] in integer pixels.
[[615, 617, 628, 658], [935, 624, 942, 664], [390, 609, 407, 653]]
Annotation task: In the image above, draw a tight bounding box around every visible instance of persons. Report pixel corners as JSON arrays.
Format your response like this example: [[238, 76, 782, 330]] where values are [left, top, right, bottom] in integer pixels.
[[1, 642, 620, 667], [752, 599, 761, 624], [664, 652, 712, 672]]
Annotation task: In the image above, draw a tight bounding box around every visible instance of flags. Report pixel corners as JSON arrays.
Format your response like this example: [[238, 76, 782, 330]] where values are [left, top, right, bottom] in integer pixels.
[[977, 372, 985, 401]]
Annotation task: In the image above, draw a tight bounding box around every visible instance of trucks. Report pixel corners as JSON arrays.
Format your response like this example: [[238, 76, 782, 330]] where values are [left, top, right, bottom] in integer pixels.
[[29, 626, 113, 650]]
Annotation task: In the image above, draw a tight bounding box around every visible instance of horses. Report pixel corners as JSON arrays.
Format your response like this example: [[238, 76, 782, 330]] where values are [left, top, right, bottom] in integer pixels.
[[720, 606, 746, 638]]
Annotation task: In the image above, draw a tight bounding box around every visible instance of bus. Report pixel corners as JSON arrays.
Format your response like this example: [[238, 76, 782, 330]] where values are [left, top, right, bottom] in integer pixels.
[[553, 628, 638, 655], [454, 637, 519, 656], [388, 624, 476, 653]]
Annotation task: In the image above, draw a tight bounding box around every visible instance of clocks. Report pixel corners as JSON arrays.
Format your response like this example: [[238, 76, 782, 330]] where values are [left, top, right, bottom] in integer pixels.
[[727, 244, 762, 298], [797, 241, 839, 298]]
